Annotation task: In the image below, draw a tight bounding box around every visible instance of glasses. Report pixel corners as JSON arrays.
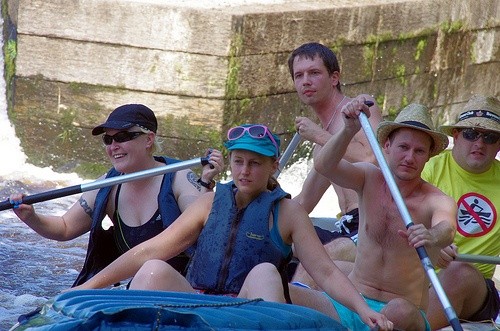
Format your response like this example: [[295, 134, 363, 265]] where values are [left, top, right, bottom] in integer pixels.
[[456, 127, 499, 144], [228, 126, 279, 152], [102, 131, 144, 146]]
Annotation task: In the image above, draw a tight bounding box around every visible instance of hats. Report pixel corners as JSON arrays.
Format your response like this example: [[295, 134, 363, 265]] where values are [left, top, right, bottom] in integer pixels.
[[377, 103, 449, 159], [439, 96, 500, 137], [224, 125, 281, 157], [92, 104, 157, 135]]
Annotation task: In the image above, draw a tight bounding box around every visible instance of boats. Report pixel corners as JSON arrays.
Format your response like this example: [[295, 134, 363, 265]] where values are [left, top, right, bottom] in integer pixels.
[[8, 216, 500, 331]]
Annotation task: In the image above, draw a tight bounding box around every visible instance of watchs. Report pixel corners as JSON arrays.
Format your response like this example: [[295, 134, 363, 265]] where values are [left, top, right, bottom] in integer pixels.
[[197, 177, 215, 189]]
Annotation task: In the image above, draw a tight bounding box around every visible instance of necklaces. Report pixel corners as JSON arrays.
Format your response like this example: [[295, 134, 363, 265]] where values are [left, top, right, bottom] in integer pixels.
[[322, 95, 347, 132]]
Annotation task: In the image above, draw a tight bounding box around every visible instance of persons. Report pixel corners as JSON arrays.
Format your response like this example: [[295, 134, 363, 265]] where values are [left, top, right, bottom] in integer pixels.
[[63, 124, 393, 331], [420, 96, 500, 330], [9, 104, 224, 294], [288, 103, 458, 331], [288, 41, 386, 292]]
